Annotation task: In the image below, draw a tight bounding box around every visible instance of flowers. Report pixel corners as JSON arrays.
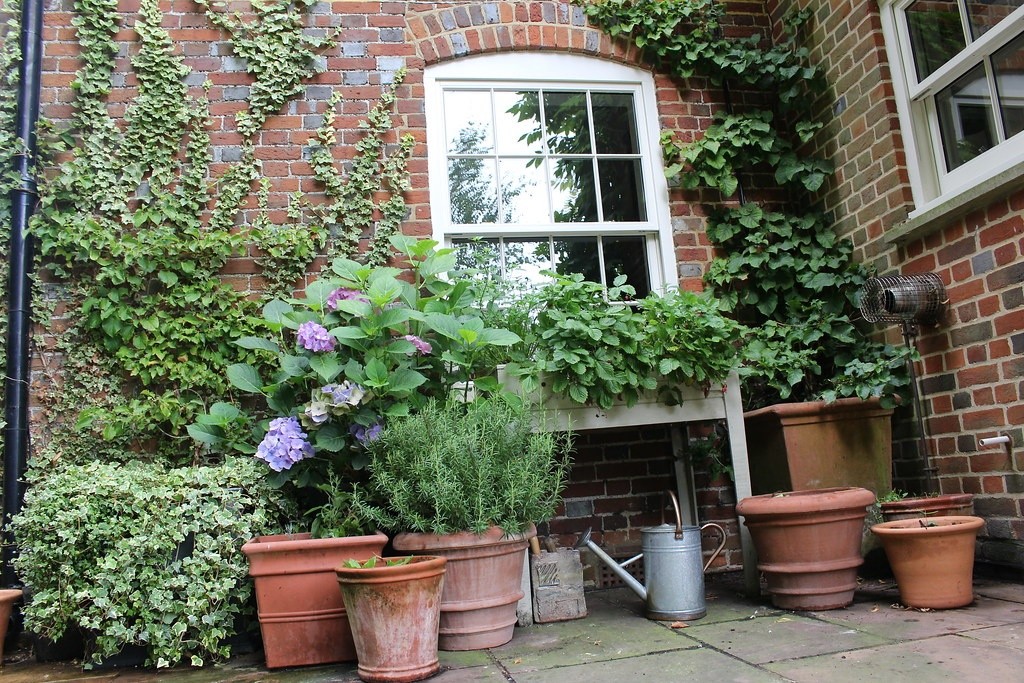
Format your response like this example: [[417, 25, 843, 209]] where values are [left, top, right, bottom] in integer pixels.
[[253, 285, 434, 475]]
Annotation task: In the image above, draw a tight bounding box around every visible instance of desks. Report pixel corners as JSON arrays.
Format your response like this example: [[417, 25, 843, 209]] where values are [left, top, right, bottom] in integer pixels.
[[452, 346, 760, 625]]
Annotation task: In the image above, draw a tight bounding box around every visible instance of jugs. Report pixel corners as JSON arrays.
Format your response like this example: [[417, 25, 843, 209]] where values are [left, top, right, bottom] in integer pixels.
[[574, 490, 725, 621]]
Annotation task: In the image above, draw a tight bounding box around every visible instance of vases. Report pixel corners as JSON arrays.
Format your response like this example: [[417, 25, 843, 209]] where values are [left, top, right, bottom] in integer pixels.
[[1, 587, 23, 665], [878, 493, 976, 521], [871, 514, 984, 609], [734, 484, 876, 610]]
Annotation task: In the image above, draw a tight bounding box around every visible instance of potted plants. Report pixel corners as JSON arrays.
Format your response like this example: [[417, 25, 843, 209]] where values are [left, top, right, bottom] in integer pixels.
[[352, 387, 574, 651], [241, 483, 389, 668], [335, 556, 451, 681]]
